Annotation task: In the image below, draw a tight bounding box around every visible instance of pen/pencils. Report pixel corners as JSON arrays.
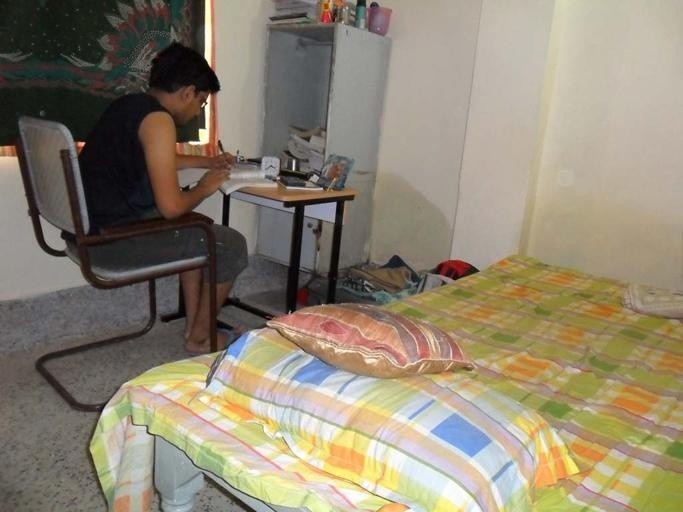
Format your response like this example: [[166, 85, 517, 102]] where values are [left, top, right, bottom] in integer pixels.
[[218, 140, 229, 164]]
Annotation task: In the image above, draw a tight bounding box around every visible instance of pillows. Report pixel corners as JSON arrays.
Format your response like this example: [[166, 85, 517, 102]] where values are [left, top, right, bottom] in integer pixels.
[[267, 302, 475, 379], [195, 326, 593, 512]]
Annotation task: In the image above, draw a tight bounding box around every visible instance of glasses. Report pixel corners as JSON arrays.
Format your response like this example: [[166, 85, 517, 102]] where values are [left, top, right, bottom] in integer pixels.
[[194, 90, 208, 109]]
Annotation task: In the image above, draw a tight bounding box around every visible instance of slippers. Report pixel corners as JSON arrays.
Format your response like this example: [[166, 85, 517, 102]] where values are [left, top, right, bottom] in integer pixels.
[[183, 329, 241, 356]]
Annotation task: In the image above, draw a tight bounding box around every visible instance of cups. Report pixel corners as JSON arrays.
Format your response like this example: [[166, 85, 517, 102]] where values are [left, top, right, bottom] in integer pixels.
[[367, 7, 393, 36]]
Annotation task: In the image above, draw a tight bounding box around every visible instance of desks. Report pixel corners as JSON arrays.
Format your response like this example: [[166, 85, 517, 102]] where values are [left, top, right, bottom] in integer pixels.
[[161, 164, 358, 335]]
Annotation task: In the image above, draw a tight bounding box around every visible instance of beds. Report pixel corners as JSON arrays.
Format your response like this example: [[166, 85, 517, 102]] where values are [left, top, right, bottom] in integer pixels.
[[88, 256, 683, 512]]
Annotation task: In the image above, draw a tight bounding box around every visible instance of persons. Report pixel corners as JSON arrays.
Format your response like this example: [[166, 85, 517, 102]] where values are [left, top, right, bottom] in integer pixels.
[[60, 42, 254, 357]]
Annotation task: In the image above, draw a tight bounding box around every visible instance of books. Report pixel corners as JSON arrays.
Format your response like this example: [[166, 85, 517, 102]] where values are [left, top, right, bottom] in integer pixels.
[[177, 162, 277, 195], [265, 0, 318, 27], [279, 126, 326, 191]]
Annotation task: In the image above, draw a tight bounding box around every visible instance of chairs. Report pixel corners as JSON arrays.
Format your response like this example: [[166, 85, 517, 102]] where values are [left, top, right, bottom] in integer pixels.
[[11, 109, 217, 412]]
